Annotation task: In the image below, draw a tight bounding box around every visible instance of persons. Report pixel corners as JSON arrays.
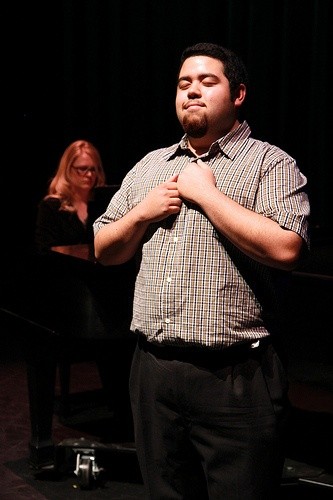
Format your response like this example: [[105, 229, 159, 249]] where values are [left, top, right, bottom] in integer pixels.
[[26, 141, 137, 475], [92, 42, 311, 499]]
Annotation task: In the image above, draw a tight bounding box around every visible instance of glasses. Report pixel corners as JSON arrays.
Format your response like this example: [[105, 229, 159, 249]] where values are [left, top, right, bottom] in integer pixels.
[[68, 163, 98, 175]]
